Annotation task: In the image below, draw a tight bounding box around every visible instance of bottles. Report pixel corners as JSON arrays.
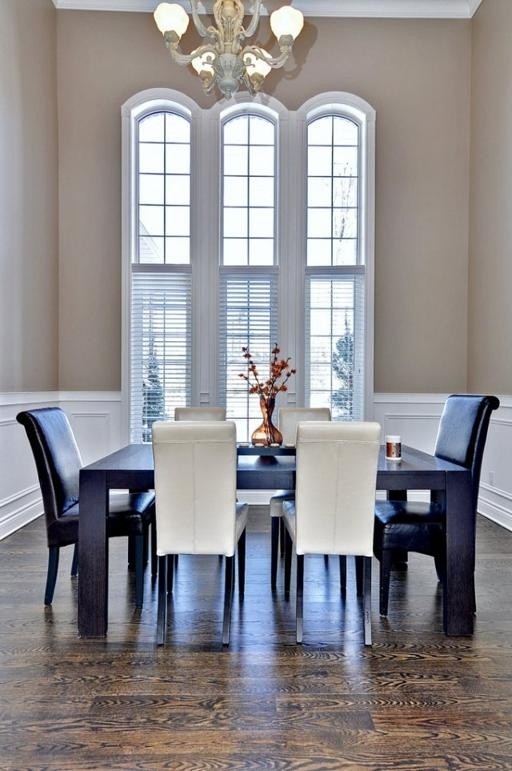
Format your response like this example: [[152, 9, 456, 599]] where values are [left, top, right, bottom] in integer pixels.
[[384, 434, 403, 462]]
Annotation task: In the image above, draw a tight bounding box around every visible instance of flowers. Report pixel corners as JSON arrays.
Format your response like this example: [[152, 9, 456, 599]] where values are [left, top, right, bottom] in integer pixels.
[[237, 341, 298, 444]]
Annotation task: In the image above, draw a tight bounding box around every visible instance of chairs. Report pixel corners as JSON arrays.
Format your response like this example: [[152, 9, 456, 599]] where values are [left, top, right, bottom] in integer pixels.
[[151, 407, 250, 646], [13, 406, 155, 623], [267, 406, 379, 640], [377, 394, 500, 640]]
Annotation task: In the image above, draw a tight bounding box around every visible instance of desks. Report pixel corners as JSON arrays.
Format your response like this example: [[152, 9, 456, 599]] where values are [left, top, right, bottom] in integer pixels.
[[74, 442, 472, 640]]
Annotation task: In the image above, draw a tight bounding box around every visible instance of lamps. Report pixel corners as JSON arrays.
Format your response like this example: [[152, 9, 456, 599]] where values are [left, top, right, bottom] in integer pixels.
[[153, 0, 307, 98]]
